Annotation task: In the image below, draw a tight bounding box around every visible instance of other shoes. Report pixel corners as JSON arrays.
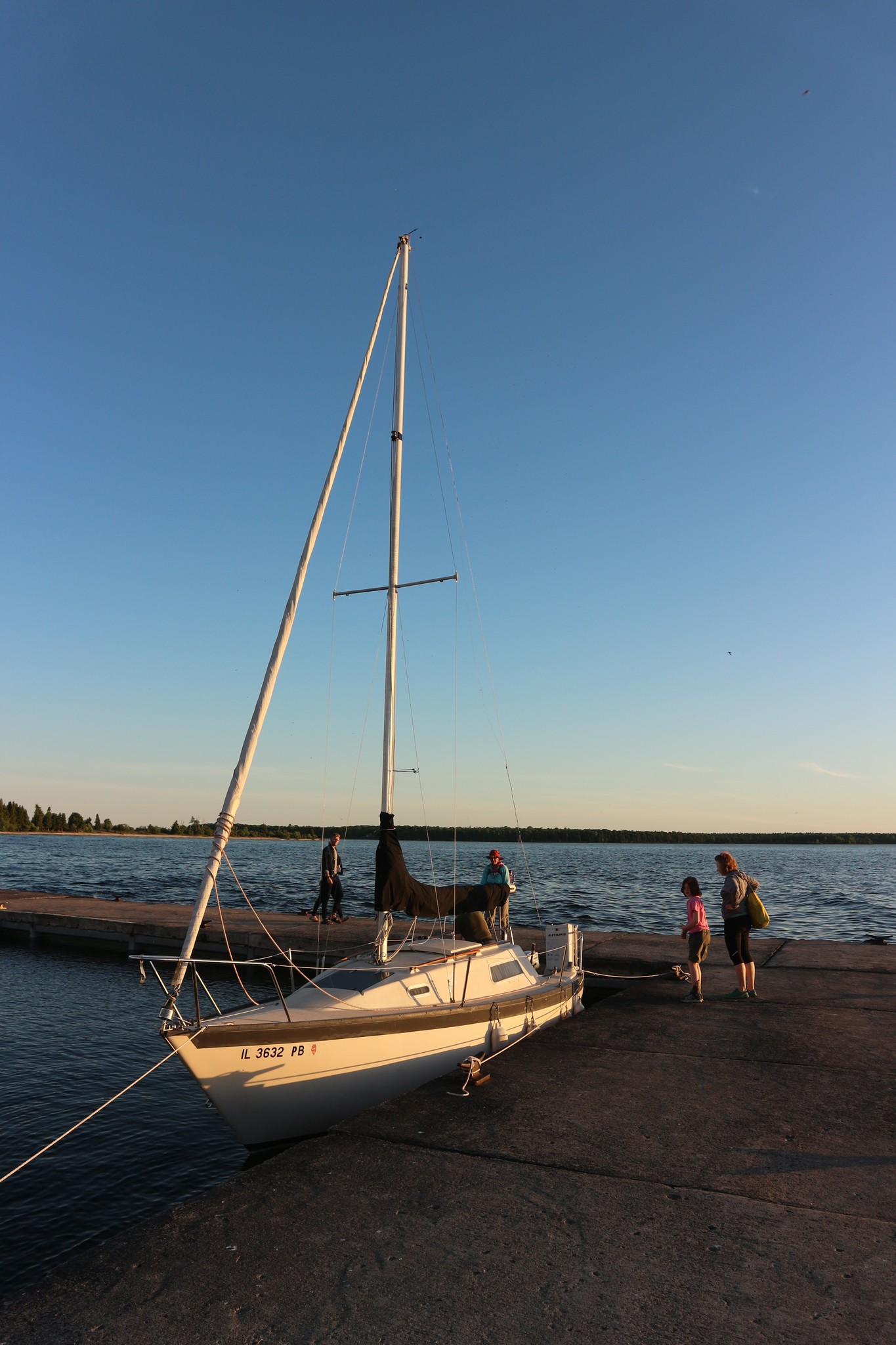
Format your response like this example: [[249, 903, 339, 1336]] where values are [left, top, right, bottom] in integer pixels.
[[501, 933, 508, 940]]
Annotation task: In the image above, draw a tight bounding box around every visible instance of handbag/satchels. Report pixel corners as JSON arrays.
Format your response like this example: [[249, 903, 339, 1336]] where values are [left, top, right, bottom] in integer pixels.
[[747, 892, 770, 929]]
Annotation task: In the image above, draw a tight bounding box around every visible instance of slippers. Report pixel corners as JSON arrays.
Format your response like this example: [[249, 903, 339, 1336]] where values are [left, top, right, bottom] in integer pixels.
[[321, 919, 334, 924], [328, 917, 343, 924], [309, 917, 321, 922], [340, 916, 350, 922]]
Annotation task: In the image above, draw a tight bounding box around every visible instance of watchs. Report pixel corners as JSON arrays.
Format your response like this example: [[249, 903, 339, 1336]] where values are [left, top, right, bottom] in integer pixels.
[[326, 876, 330, 878]]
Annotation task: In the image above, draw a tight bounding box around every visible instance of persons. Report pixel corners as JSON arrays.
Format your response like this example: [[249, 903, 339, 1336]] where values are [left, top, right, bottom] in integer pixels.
[[321, 833, 344, 924], [309, 841, 350, 922], [481, 850, 510, 940], [679, 877, 711, 1002], [714, 851, 760, 999], [453, 911, 496, 946]]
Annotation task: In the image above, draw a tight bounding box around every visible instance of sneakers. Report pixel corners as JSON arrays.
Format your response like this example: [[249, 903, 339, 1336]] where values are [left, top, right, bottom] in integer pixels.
[[680, 993, 703, 1002], [747, 989, 757, 996], [725, 988, 749, 998]]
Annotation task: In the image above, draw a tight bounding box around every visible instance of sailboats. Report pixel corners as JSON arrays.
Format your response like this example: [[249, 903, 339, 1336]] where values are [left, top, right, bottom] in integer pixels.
[[128, 226, 587, 1161]]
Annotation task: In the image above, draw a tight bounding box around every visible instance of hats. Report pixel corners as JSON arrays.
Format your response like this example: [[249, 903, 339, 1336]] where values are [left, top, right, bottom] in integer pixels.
[[486, 850, 504, 860]]
